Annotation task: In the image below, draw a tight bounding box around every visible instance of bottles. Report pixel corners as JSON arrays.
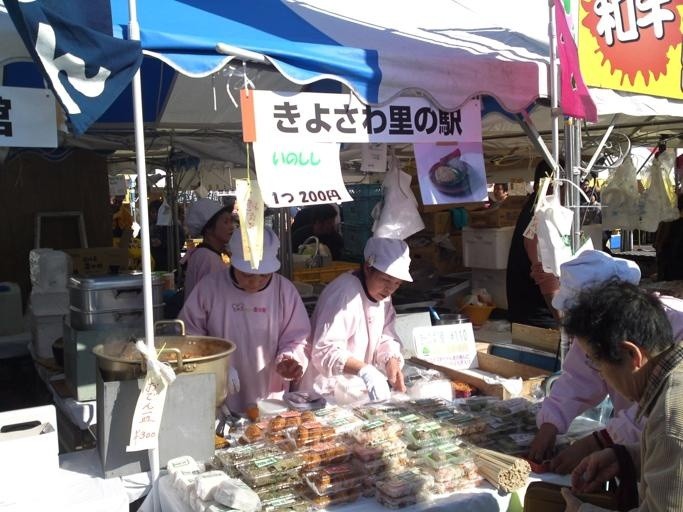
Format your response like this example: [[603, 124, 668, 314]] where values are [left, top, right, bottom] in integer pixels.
[[364, 237, 413, 282], [551, 250, 642, 311], [229, 225, 281, 274], [186, 198, 223, 235]]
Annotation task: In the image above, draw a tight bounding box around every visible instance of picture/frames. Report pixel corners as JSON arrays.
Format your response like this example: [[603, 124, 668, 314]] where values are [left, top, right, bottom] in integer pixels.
[[156, 395, 607, 511]]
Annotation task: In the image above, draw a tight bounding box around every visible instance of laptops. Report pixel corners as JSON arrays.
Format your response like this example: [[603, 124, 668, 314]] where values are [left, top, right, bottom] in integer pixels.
[[430, 148, 480, 197]]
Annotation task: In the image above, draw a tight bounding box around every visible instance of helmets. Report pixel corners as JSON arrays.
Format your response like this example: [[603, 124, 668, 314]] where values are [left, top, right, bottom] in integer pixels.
[[283, 392, 326, 413]]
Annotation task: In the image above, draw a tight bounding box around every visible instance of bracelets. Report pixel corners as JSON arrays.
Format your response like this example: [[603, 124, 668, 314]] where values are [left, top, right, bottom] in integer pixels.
[[458, 299, 496, 328]]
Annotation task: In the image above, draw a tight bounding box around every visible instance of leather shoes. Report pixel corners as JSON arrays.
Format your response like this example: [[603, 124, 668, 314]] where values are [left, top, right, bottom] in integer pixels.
[[228, 365, 240, 394], [358, 365, 390, 401]]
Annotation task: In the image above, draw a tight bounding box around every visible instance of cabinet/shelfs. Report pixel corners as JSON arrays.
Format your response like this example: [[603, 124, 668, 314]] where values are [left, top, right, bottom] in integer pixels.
[[610, 228, 623, 255]]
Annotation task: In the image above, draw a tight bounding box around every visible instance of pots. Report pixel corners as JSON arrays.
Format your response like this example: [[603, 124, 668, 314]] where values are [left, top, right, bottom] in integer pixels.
[[91, 320, 239, 407], [66, 272, 175, 331]]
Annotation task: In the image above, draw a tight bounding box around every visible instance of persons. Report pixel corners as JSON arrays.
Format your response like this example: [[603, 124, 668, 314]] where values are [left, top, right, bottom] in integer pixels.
[[527, 248, 683, 476], [580, 179, 603, 225], [652, 191, 683, 282], [112, 193, 124, 238], [505, 158, 565, 332], [178, 206, 236, 305], [291, 237, 414, 401], [175, 221, 313, 423], [285, 204, 346, 262], [148, 200, 185, 271], [489, 183, 509, 209], [560, 280, 683, 512]]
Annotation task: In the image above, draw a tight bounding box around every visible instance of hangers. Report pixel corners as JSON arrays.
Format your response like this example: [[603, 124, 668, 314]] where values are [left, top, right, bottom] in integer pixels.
[[293, 390, 318, 403]]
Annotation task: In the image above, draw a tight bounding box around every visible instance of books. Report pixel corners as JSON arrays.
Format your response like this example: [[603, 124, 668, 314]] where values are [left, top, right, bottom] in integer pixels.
[[462, 225, 516, 310], [0, 281, 24, 334], [65, 270, 166, 331], [28, 248, 68, 359]]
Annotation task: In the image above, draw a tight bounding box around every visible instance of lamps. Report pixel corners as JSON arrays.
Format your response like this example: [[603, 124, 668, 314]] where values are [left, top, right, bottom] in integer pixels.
[[0, 404, 86, 511]]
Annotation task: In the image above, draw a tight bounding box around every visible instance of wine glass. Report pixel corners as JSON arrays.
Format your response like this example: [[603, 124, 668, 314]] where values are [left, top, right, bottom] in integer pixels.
[[574, 468, 583, 475]]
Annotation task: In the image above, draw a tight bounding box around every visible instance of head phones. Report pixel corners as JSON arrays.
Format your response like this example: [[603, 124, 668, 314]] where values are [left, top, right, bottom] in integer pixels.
[[469, 443, 531, 494]]
